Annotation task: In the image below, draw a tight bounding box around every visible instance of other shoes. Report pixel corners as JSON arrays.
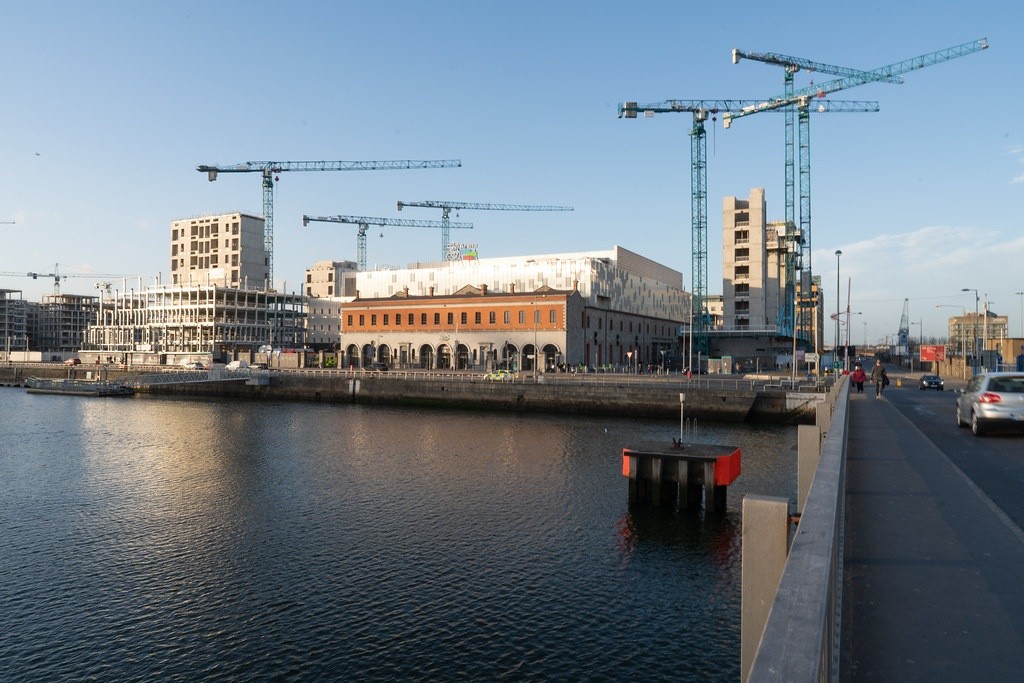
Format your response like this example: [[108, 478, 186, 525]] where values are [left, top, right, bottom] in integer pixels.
[[879, 395, 882, 399], [861, 390, 863, 393], [876, 395, 879, 399], [857, 391, 861, 393]]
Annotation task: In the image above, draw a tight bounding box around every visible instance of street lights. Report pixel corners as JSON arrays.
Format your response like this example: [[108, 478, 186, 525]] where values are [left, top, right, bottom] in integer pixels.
[[834, 250, 844, 356], [935, 288, 979, 359], [911, 322, 922, 371]]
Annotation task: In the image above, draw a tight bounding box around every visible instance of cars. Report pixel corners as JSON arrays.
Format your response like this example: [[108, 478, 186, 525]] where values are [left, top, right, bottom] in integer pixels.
[[369, 362, 389, 373], [852, 356, 866, 366], [183, 362, 270, 372], [953, 371, 1024, 436], [918, 375, 944, 391], [64, 358, 81, 365], [682, 367, 707, 376]]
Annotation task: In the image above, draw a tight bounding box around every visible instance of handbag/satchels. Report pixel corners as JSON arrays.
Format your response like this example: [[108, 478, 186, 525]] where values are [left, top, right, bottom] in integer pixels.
[[852, 381, 856, 388]]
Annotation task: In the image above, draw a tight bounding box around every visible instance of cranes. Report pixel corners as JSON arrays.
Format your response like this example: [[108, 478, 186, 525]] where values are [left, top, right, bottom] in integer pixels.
[[395, 200, 575, 262], [196, 158, 461, 311], [302, 215, 475, 271], [721, 34, 988, 340], [617, 98, 882, 362], [0, 263, 135, 296], [731, 49, 902, 332]]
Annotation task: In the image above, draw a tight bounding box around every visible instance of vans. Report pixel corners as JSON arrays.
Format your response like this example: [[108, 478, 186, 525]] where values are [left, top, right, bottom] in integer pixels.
[[225, 360, 248, 372]]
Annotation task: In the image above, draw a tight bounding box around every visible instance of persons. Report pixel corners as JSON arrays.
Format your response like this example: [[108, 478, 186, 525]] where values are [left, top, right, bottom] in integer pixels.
[[869, 360, 885, 399], [647, 364, 658, 373], [557, 361, 588, 373], [787, 361, 790, 369], [851, 363, 867, 393]]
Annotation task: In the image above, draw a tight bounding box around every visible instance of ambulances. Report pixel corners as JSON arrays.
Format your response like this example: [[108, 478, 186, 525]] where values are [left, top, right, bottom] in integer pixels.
[[312, 353, 337, 367], [483, 370, 515, 382]]
